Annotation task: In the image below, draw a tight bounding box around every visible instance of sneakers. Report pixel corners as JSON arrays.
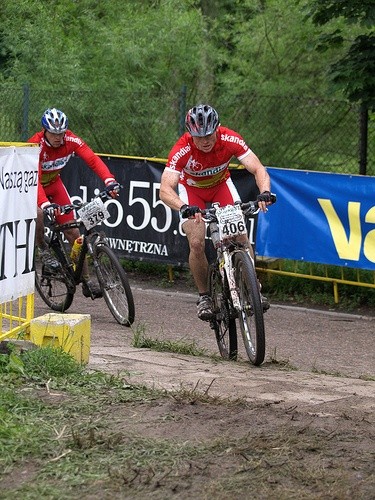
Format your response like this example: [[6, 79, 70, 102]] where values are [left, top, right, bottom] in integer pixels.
[[196, 294, 214, 320], [82, 280, 102, 297], [37, 244, 62, 272]]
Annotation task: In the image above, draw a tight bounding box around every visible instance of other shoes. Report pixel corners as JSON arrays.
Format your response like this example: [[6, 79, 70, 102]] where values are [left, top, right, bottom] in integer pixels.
[[247, 283, 270, 308]]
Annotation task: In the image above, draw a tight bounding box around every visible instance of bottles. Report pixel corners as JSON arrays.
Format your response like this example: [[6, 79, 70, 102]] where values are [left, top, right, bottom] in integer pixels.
[[69, 237, 83, 262]]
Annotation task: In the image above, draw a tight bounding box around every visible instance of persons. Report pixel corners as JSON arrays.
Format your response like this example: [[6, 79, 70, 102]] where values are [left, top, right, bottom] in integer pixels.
[[159, 105, 273, 320], [26, 107, 122, 297]]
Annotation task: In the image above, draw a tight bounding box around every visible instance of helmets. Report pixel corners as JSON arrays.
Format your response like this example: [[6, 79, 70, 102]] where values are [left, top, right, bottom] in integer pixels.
[[41, 107, 68, 134], [184, 104, 220, 138]]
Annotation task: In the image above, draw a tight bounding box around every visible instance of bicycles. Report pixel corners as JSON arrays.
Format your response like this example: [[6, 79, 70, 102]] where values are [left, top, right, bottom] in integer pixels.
[[31, 184, 135, 327], [198, 199, 270, 367]]
